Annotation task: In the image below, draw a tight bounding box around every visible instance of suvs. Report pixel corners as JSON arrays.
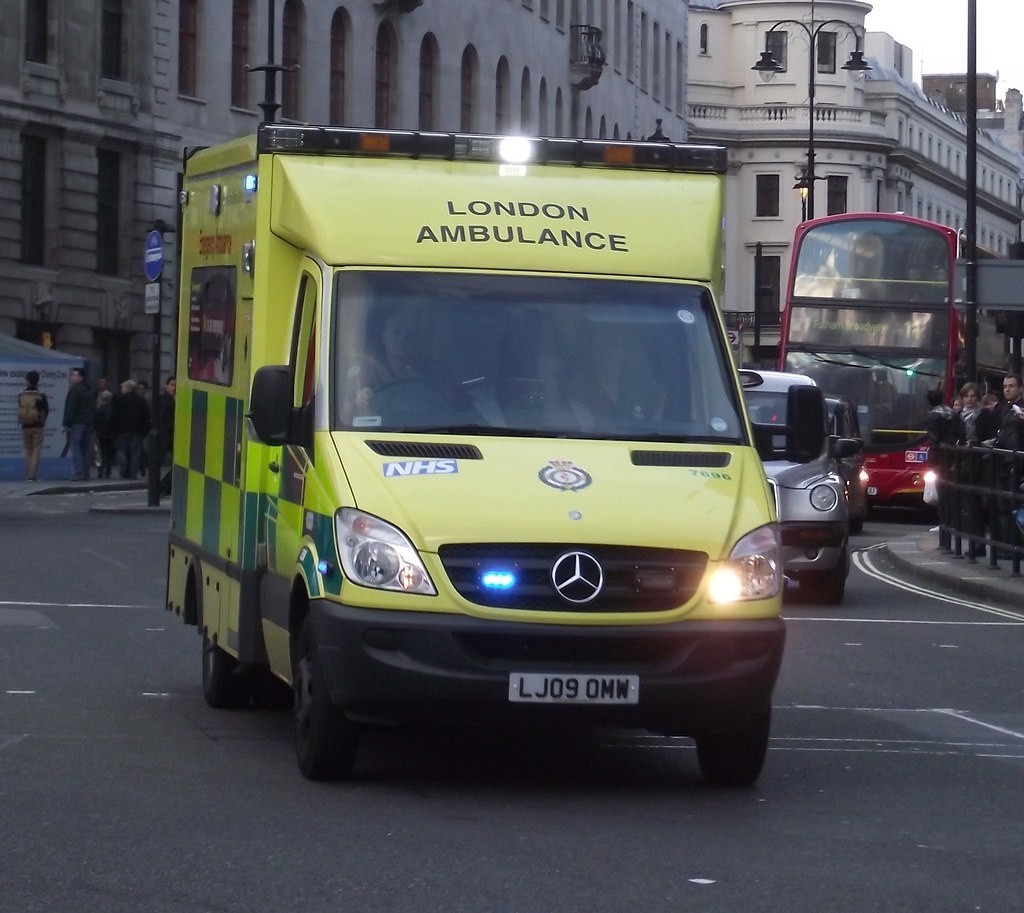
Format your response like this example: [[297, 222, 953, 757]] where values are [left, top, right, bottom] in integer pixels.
[[738, 367, 855, 602]]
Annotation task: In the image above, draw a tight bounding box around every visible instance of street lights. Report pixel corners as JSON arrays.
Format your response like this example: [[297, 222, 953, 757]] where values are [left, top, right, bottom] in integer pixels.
[[748, 0, 872, 226], [788, 166, 814, 225]]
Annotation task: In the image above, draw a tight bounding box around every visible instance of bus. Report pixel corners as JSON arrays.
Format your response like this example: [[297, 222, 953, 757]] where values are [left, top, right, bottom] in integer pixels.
[[776, 210, 1001, 513]]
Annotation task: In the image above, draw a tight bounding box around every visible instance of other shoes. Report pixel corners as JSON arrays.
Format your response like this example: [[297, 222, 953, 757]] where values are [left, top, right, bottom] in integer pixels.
[[105, 472, 111, 478], [71, 476, 85, 481], [118, 471, 129, 479], [965, 547, 987, 556], [25, 476, 39, 482], [98, 472, 103, 479]]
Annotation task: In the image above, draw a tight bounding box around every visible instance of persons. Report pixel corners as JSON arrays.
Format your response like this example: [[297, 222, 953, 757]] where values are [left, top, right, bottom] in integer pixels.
[[926, 374, 1024, 557], [63, 367, 176, 482], [18, 371, 49, 483], [342, 307, 421, 419], [908, 265, 949, 304]]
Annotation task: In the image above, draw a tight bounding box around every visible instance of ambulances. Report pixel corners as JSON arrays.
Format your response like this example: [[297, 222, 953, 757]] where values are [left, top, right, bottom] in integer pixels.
[[169, 126, 792, 787]]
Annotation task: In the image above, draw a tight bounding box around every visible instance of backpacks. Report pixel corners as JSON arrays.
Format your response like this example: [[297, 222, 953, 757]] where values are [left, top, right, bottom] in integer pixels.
[[19, 390, 44, 425]]
